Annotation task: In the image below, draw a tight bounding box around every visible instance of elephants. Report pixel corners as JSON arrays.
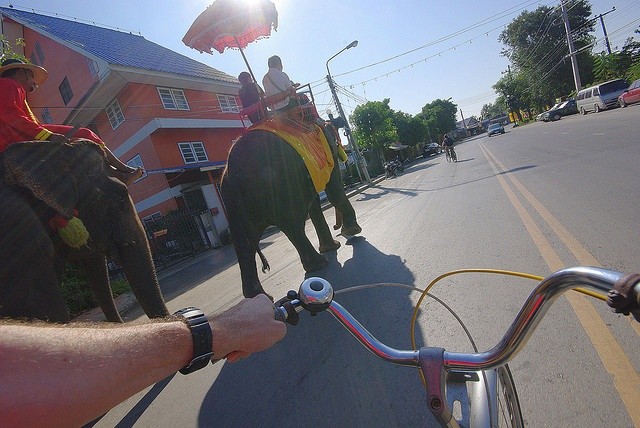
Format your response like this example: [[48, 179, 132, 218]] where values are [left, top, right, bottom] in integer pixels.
[[220, 118, 361, 304], [0, 138, 169, 324]]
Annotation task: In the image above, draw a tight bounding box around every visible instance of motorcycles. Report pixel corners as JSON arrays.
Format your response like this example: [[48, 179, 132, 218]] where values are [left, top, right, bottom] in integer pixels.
[[384, 155, 404, 178], [422, 144, 432, 159]]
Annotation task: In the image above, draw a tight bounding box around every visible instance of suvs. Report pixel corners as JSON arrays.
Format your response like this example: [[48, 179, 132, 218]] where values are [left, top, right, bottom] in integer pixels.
[[536, 102, 563, 122], [544, 99, 579, 123]]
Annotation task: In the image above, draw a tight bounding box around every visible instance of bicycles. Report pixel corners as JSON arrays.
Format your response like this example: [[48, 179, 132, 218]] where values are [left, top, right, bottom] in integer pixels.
[[211, 264, 639, 427], [444, 147, 458, 163]]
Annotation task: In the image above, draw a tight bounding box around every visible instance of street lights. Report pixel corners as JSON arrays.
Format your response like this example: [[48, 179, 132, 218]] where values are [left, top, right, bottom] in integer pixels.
[[326, 40, 375, 184]]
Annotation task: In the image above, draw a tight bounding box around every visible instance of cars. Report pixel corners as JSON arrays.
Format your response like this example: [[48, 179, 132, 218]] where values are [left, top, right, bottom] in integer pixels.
[[618, 78, 640, 108], [486, 122, 505, 137], [426, 142, 441, 156]]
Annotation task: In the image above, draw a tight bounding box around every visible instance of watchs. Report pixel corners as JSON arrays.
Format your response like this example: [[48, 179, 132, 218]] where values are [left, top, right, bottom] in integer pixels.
[[172, 305, 214, 375]]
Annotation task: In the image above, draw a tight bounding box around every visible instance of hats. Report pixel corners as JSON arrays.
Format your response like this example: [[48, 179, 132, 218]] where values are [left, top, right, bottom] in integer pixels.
[[0, 59, 48, 85]]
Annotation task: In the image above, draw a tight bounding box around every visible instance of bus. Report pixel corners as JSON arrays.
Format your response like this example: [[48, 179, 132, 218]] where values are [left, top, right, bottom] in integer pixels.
[[576, 77, 630, 115]]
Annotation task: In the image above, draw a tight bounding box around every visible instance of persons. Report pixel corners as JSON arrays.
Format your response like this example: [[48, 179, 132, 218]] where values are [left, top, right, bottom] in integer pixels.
[[0, 292, 288, 428], [441, 135, 455, 161], [237, 70, 270, 125], [0, 58, 146, 186], [261, 55, 315, 123]]
